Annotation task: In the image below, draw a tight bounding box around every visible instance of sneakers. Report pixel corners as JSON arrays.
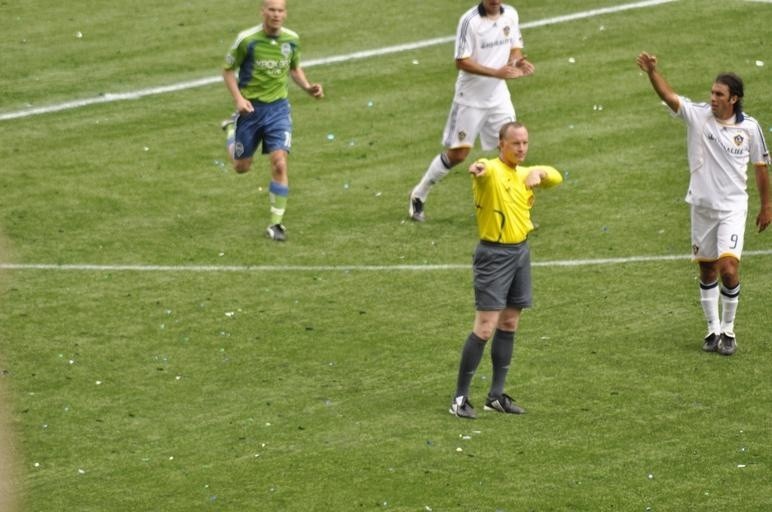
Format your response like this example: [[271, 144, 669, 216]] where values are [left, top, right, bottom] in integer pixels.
[[221, 110, 239, 131], [449, 398, 477, 420], [702, 332, 717, 352], [265, 222, 288, 242], [717, 332, 736, 355], [408, 189, 427, 223], [484, 394, 525, 414]]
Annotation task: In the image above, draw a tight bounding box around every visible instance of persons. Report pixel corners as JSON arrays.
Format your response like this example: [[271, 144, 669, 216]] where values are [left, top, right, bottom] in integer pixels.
[[409, 0, 535, 222], [449, 119, 564, 419], [219, 0, 325, 241], [635, 49, 771, 356]]
